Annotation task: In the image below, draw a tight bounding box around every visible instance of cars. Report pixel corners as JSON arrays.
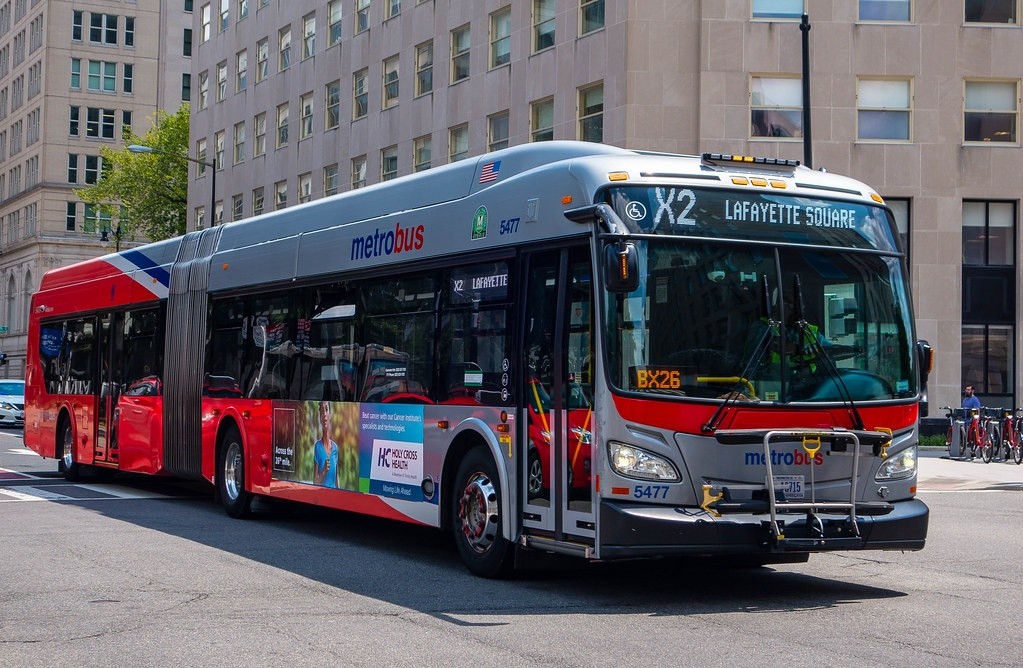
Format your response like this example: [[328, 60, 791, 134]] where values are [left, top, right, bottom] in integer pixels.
[[381, 394, 574, 499], [0, 379, 25, 427], [526, 376, 593, 488]]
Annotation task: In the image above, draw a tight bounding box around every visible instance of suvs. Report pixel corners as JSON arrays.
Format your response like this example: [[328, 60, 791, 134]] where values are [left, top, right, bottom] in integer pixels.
[[110, 375, 242, 458]]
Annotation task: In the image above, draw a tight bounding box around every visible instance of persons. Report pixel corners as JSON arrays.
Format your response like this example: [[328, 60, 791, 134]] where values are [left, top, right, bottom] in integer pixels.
[[961, 384, 980, 426], [313, 402, 339, 489], [747, 294, 856, 381]]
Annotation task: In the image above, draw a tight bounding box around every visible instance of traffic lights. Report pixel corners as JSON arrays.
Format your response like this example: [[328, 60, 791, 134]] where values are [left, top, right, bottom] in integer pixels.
[[828, 297, 857, 338], [0, 353, 7, 366]]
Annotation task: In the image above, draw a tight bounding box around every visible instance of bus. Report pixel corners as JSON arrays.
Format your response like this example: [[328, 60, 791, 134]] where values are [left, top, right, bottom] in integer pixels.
[[23, 140, 934, 577]]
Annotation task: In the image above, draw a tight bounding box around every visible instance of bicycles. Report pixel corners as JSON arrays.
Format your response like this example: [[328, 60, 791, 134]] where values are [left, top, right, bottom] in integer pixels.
[[939, 406, 1023, 465]]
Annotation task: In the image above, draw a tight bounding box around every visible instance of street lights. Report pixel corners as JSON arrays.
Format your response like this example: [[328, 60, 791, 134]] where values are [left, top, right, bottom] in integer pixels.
[[100, 225, 120, 252], [127, 145, 216, 228]]
